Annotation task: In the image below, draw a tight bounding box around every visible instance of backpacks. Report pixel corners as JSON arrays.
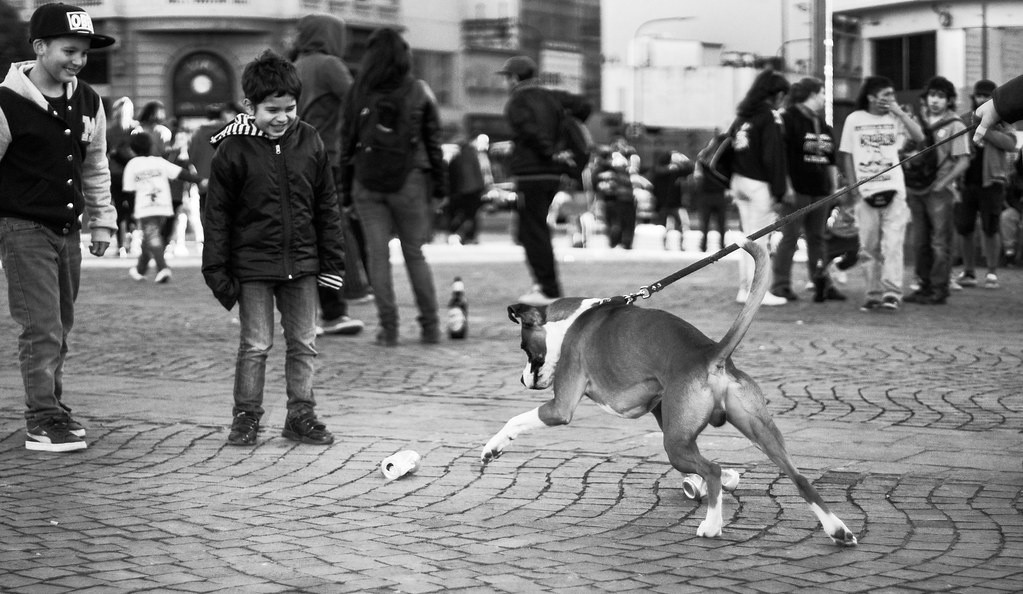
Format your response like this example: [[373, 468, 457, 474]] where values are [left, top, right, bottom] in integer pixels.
[[896, 113, 963, 190]]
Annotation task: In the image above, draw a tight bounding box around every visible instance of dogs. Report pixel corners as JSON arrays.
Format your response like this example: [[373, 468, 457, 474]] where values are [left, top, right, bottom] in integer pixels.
[[480, 234, 863, 547]]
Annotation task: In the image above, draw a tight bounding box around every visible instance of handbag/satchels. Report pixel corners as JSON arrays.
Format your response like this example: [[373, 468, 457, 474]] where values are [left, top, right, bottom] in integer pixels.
[[699, 136, 735, 186], [864, 192, 896, 209]]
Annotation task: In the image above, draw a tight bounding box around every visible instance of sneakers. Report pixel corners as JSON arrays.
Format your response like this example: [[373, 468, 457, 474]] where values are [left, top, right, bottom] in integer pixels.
[[53, 413, 86, 439], [25, 423, 88, 452], [228, 410, 260, 446], [281, 412, 335, 445]]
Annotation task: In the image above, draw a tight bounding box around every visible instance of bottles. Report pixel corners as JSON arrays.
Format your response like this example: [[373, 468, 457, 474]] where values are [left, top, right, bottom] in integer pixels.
[[447, 276, 467, 339]]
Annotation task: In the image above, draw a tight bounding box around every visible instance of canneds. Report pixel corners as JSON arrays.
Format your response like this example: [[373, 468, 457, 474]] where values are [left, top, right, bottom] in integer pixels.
[[381, 450, 420, 479]]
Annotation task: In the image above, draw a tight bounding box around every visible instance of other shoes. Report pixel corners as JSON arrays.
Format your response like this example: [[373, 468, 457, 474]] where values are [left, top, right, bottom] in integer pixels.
[[880, 294, 899, 308], [860, 300, 880, 311], [947, 278, 963, 290], [129, 266, 144, 282], [813, 286, 846, 303], [321, 317, 362, 336], [519, 287, 558, 306], [902, 289, 947, 305], [955, 272, 978, 285], [984, 273, 999, 289], [767, 283, 799, 301], [374, 329, 396, 344], [154, 270, 172, 282]]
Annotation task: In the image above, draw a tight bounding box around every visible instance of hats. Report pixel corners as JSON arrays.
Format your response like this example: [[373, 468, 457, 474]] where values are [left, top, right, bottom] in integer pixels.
[[28, 3, 116, 50], [973, 78, 996, 95], [494, 55, 536, 78]]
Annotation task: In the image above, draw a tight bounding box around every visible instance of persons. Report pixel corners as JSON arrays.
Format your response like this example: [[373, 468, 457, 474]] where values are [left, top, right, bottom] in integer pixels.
[[952, 80, 1018, 290], [646, 149, 699, 243], [103, 96, 240, 284], [0, 2, 119, 455], [338, 26, 450, 346], [730, 68, 848, 306], [838, 76, 930, 313], [592, 151, 638, 249], [969, 74, 1023, 150], [442, 134, 493, 246], [903, 75, 972, 305], [201, 49, 348, 447], [285, 15, 357, 334], [999, 148, 1023, 269], [487, 55, 596, 307]]
